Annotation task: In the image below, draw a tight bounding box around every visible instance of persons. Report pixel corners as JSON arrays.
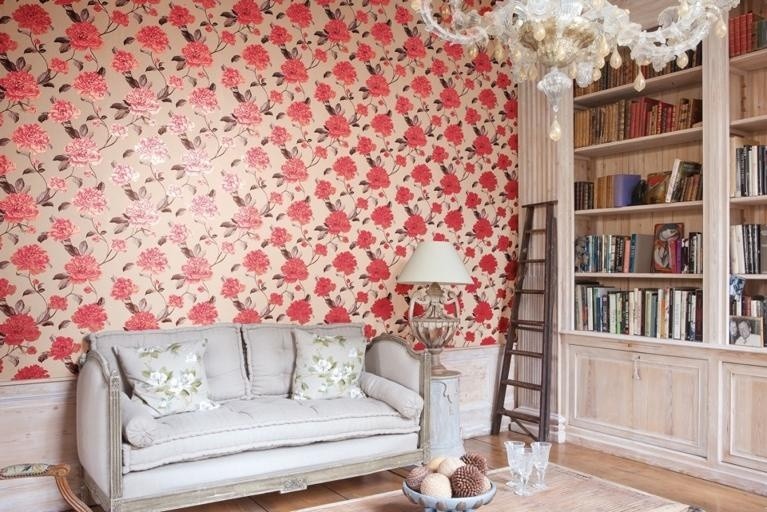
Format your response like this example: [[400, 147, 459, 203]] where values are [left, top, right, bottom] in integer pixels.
[[730, 318, 761, 345]]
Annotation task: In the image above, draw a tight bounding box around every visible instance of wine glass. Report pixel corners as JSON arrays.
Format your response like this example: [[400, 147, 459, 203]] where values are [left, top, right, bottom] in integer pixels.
[[502, 439, 552, 497]]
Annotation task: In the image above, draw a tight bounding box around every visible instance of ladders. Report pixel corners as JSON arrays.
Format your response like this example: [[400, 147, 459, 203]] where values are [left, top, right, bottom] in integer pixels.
[[491, 200, 557, 442]]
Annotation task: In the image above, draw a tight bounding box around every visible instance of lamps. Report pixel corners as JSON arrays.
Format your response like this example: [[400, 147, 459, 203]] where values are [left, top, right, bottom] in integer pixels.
[[396, 242, 475, 376], [417, 1, 742, 145]]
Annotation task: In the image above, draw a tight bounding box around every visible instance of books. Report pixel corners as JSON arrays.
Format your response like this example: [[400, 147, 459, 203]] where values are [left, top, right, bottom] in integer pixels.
[[574, 11, 767, 97], [730, 223, 766, 318], [575, 160, 704, 210], [574, 223, 702, 342], [575, 96, 700, 148], [732, 143, 767, 198]]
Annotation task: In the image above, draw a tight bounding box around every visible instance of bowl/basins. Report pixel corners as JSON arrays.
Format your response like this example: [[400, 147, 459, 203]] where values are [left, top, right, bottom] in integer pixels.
[[401, 480, 497, 511]]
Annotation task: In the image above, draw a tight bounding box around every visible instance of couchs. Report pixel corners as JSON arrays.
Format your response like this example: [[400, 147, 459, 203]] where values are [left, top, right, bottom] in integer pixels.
[[77, 322, 426, 512]]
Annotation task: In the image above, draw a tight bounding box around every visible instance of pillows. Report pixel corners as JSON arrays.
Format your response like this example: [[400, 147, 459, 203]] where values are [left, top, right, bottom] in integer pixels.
[[113, 337, 220, 417], [288, 327, 368, 400]]
